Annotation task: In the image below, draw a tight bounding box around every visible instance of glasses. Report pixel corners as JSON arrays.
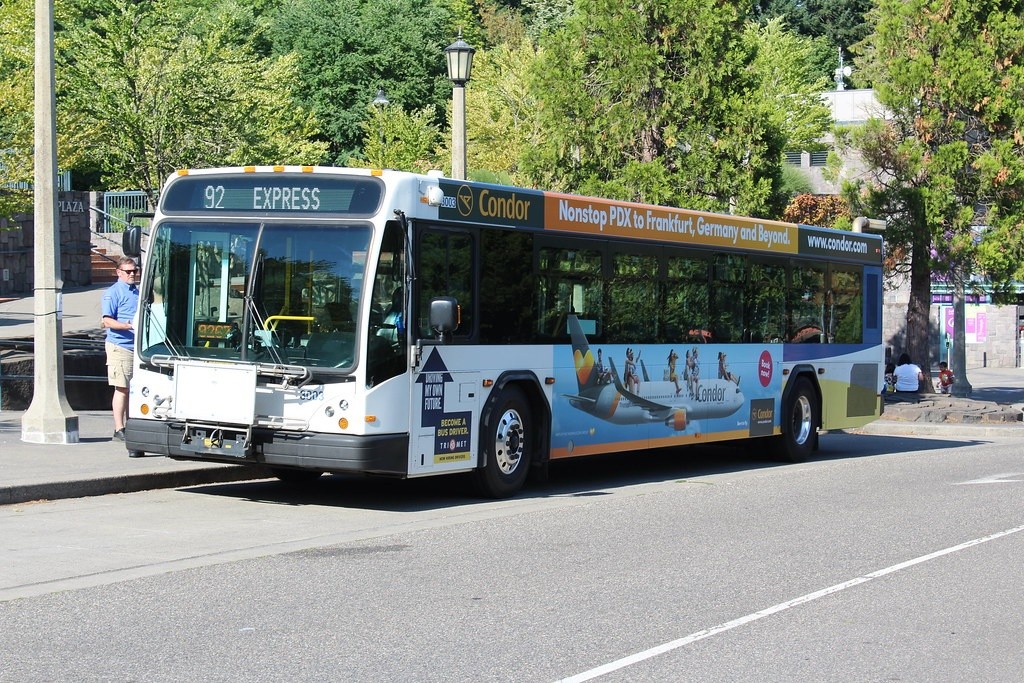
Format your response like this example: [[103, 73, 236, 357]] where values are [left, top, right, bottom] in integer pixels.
[[122, 270, 137, 274]]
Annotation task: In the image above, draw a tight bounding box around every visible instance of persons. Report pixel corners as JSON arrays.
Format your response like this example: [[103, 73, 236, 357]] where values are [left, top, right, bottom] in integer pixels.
[[884, 364, 897, 393], [102, 258, 140, 442], [893, 352, 924, 393], [597, 347, 741, 395], [936, 361, 955, 394]]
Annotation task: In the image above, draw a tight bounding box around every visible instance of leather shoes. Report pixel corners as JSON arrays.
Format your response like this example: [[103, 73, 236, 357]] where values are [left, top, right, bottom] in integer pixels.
[[112, 427, 126, 442]]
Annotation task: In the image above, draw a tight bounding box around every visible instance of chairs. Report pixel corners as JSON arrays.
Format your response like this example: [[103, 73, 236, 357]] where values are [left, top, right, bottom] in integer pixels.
[[376, 286, 407, 346], [321, 302, 354, 333]]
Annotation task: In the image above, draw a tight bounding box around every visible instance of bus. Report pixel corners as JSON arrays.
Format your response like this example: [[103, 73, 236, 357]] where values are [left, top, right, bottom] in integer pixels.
[[122, 157, 886, 502]]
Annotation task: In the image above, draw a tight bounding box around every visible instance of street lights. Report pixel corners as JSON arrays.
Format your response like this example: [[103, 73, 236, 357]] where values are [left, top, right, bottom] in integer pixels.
[[372, 89, 391, 168], [443, 25, 476, 180]]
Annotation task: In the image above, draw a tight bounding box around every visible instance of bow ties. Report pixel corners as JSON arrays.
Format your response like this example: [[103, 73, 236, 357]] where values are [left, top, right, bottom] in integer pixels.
[[129, 284, 136, 291]]
